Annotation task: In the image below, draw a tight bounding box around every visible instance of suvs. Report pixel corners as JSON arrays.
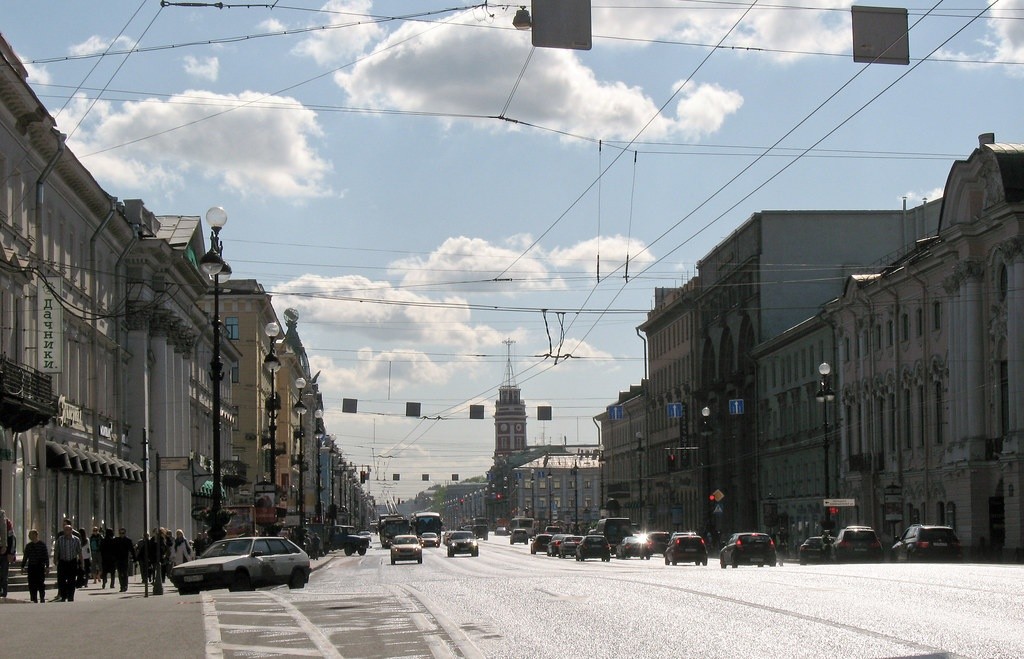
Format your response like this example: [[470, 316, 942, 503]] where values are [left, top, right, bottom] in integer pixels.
[[890, 524, 963, 563]]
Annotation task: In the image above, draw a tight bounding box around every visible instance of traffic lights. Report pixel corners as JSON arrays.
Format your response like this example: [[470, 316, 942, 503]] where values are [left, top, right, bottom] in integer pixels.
[[709, 495, 715, 512]]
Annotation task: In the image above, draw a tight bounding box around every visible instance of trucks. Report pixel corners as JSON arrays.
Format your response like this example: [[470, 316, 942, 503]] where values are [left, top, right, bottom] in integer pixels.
[[471, 517, 489, 540], [588, 518, 633, 555], [305, 524, 369, 556]]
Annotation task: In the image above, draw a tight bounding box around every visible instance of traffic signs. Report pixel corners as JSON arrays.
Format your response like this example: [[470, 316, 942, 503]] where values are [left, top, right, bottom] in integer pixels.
[[824, 499, 855, 506]]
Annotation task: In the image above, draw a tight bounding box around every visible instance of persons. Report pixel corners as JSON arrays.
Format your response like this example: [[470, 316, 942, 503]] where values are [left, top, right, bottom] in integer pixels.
[[0, 509, 17, 596], [304, 533, 321, 560], [703, 529, 719, 552], [21, 530, 49, 603], [822, 532, 832, 563], [54, 519, 84, 602], [79, 527, 208, 592]]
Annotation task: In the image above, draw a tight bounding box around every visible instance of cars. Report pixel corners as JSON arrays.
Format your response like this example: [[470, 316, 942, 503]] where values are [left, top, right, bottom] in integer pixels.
[[171, 536, 311, 595], [496, 526, 884, 568], [358, 531, 479, 565]]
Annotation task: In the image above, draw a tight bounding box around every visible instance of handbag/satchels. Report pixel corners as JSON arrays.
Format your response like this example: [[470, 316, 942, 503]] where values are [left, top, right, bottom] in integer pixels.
[[303, 540, 308, 545], [76, 573, 85, 587], [135, 545, 141, 561]]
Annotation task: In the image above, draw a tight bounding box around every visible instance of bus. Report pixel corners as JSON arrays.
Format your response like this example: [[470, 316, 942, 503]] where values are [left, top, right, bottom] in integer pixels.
[[377, 512, 442, 549], [510, 516, 534, 537]]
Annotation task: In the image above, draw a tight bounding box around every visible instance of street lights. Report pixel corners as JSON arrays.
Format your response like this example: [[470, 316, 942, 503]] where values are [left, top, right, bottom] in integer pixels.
[[329, 434, 353, 526], [699, 406, 714, 524], [264, 322, 282, 484], [814, 363, 835, 529], [294, 377, 308, 525], [450, 444, 606, 531], [200, 206, 233, 540], [313, 409, 324, 522], [634, 432, 645, 523]]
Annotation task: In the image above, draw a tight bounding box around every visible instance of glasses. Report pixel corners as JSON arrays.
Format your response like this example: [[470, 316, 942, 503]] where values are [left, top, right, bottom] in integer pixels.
[[119, 532, 125, 535]]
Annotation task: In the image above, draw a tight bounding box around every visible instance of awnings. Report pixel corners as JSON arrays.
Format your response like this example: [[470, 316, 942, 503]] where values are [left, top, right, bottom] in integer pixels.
[[46, 441, 143, 483], [191, 481, 225, 499]]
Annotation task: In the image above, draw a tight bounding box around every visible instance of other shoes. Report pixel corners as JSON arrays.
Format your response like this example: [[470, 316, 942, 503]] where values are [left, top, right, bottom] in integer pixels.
[[119, 589, 127, 592], [102, 585, 105, 588], [110, 585, 115, 588], [98, 579, 101, 582], [61, 596, 67, 602], [93, 581, 96, 584], [68, 598, 74, 602]]
